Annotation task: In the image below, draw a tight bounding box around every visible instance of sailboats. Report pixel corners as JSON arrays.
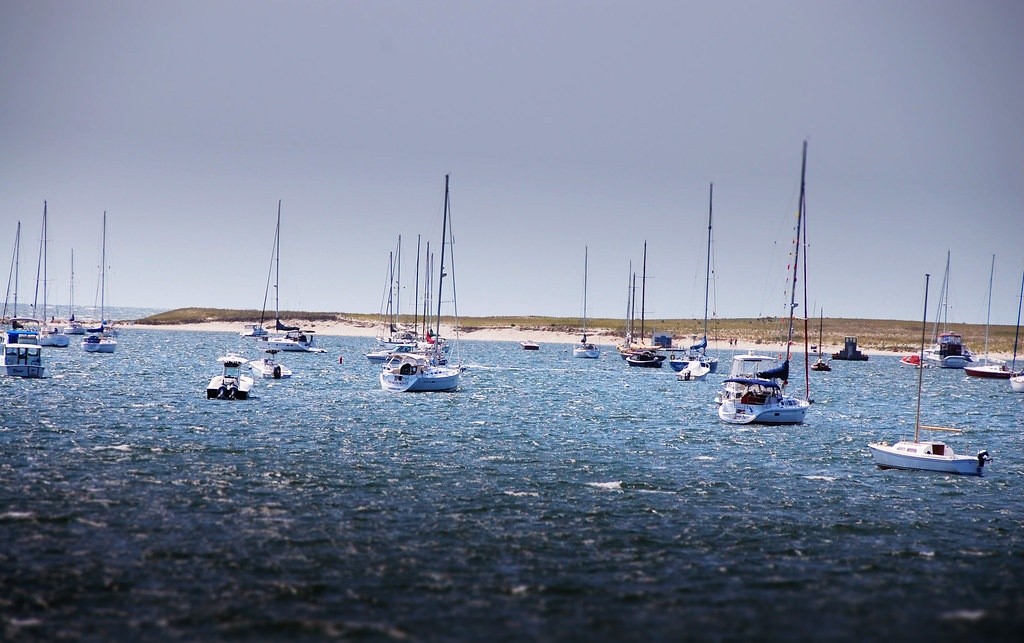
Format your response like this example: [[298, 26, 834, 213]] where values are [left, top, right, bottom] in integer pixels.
[[573, 246, 600, 358], [868, 251, 1024, 477], [368, 173, 466, 393], [715, 140, 817, 424], [0, 199, 117, 380], [616, 183, 719, 383], [810, 309, 833, 373]]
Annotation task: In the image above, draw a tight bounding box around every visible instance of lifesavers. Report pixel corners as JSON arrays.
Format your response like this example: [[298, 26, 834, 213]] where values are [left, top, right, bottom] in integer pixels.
[[400, 363, 411, 375], [940, 343, 946, 348], [670, 354, 675, 360]]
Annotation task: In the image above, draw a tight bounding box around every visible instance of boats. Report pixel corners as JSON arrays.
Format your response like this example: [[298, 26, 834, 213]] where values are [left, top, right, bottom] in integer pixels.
[[241, 199, 326, 354], [831, 335, 870, 360], [206, 354, 253, 399], [250, 348, 292, 379], [522, 341, 540, 349]]
